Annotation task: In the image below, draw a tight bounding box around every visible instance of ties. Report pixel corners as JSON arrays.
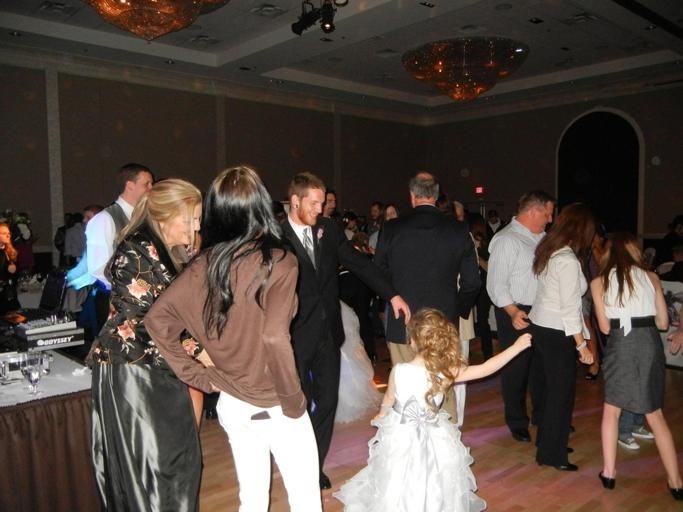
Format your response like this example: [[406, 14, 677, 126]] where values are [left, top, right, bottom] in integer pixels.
[[302, 229, 316, 269]]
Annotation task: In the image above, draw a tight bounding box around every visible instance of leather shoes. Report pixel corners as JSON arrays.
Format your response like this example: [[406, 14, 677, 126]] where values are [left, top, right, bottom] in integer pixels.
[[598, 470, 614, 488], [584, 364, 600, 381], [509, 427, 531, 442], [536, 424, 579, 470], [205, 408, 217, 420], [667, 483, 683, 500], [319, 473, 331, 489]]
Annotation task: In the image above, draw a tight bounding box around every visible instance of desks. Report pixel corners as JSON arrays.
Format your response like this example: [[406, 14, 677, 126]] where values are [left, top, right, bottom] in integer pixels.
[[16, 281, 90, 360], [0, 346, 104, 510]]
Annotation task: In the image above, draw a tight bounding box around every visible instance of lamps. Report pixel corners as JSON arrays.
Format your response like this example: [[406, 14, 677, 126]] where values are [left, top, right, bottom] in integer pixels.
[[402, 38, 530, 106], [290, 0, 349, 38], [81, 1, 230, 44]]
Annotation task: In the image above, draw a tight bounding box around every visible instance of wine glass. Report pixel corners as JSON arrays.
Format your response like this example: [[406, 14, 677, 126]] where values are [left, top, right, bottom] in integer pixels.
[[20, 352, 31, 392], [26, 353, 45, 395], [17, 270, 50, 293]]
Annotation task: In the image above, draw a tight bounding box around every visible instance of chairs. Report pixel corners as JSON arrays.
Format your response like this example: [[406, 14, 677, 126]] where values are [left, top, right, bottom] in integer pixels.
[[15, 267, 67, 323]]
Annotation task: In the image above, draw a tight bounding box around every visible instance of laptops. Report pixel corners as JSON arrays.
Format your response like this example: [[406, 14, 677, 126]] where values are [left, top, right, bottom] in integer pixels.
[[1, 267, 67, 327]]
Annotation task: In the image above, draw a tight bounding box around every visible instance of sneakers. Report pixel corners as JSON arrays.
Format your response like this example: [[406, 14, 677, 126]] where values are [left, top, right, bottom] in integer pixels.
[[618, 426, 653, 451]]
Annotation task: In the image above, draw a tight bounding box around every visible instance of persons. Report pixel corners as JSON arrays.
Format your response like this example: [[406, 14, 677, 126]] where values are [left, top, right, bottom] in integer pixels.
[[57, 161, 682, 453], [0, 221, 21, 315], [587, 227, 682, 503], [11, 213, 40, 273], [276, 168, 414, 489], [82, 178, 208, 512], [527, 199, 595, 472], [329, 308, 535, 512], [146, 165, 329, 512]]
